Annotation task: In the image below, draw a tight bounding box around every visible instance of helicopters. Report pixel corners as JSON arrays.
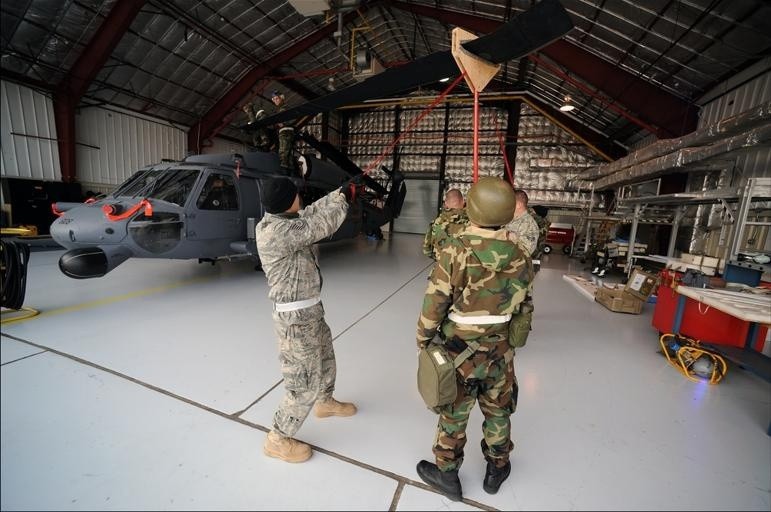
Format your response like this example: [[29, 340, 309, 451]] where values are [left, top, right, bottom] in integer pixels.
[[50, 0, 576, 279]]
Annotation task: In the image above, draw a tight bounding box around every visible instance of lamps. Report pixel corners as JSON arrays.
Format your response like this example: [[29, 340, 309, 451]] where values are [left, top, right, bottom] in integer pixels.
[[559, 70, 575, 112]]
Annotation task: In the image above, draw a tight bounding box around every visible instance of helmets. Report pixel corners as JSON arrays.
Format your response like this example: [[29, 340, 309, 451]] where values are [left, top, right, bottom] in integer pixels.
[[467, 176, 516, 228]]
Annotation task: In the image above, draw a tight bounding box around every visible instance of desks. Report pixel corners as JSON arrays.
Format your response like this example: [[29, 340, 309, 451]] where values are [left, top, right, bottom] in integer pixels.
[[667, 283, 770, 351]]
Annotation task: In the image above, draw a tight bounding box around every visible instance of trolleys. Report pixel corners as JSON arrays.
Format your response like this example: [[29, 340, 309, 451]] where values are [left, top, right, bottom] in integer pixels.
[[541, 225, 574, 255]]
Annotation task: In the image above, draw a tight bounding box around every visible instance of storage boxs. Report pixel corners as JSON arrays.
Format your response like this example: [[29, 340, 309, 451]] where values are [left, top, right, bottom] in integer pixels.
[[594, 268, 659, 317], [653, 264, 771, 358]]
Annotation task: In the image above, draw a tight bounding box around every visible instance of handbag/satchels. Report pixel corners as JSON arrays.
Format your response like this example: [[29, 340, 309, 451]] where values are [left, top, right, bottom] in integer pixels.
[[418, 346, 458, 408], [507, 312, 530, 348]]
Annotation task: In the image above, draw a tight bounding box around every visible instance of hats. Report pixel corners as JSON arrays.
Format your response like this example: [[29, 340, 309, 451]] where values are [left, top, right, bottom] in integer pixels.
[[259, 176, 298, 214]]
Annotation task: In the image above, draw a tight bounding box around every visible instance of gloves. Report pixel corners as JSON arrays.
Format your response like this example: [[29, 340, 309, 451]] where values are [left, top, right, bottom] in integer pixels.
[[338, 176, 366, 204]]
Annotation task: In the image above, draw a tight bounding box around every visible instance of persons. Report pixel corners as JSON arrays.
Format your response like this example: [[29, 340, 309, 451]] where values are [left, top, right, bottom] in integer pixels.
[[417, 176, 534, 502], [242, 86, 299, 175], [254, 174, 366, 464], [422, 188, 551, 277]]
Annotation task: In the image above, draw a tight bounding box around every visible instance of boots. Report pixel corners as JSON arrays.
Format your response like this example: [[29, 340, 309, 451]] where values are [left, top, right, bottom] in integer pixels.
[[315, 398, 357, 418], [416, 460, 463, 501], [483, 460, 511, 494], [264, 432, 313, 463]]
